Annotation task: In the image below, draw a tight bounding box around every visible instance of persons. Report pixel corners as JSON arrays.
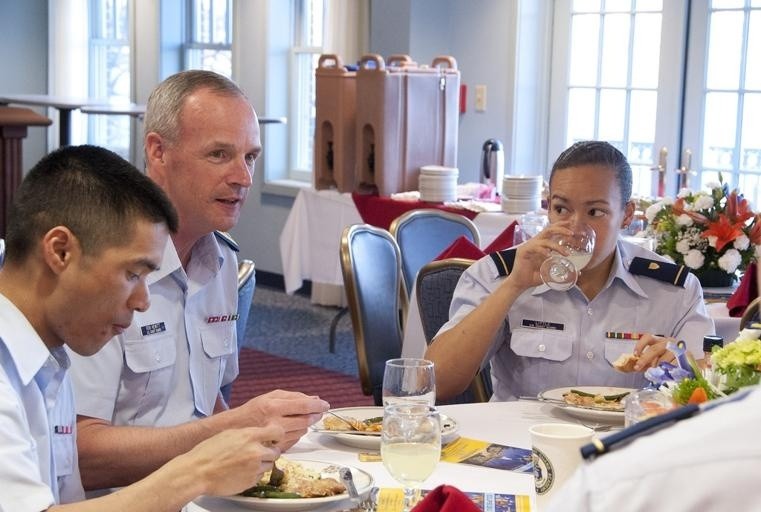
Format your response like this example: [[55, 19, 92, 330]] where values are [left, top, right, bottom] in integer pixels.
[[0, 144, 288, 512], [64, 68, 337, 491], [419, 139, 724, 401], [539, 381, 761, 512]]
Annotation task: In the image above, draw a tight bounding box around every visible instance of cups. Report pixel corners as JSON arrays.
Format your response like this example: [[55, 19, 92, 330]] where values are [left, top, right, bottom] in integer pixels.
[[534, 424, 592, 508]]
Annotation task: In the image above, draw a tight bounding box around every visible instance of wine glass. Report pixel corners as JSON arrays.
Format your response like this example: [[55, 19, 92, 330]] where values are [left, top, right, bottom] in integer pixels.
[[381, 412, 439, 506], [384, 358, 437, 411], [541, 222, 598, 293]]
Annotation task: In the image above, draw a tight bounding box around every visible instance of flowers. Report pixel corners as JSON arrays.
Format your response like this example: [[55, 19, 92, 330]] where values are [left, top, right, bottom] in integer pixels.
[[639, 170, 761, 274], [710, 339, 761, 387]]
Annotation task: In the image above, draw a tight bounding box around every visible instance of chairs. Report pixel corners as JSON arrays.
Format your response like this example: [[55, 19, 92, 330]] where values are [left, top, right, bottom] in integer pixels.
[[218, 260, 256, 407], [740, 297, 760, 340], [339, 207, 493, 405]]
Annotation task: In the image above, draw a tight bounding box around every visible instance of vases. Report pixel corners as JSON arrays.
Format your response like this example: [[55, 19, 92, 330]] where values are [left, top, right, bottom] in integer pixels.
[[692, 265, 734, 287]]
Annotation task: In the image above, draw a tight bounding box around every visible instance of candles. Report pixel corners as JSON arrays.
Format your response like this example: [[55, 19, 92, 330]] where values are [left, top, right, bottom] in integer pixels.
[[685, 148, 692, 170], [659, 146, 668, 173]]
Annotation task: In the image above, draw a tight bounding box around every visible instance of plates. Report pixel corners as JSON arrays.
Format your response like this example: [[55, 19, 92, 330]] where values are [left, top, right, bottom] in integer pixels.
[[212, 458, 374, 506], [502, 175, 545, 212], [539, 387, 677, 421], [419, 165, 461, 203], [307, 407, 460, 449]]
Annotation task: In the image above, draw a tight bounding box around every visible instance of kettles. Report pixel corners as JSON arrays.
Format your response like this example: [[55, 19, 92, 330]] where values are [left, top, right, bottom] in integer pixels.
[[477, 138, 506, 192]]
